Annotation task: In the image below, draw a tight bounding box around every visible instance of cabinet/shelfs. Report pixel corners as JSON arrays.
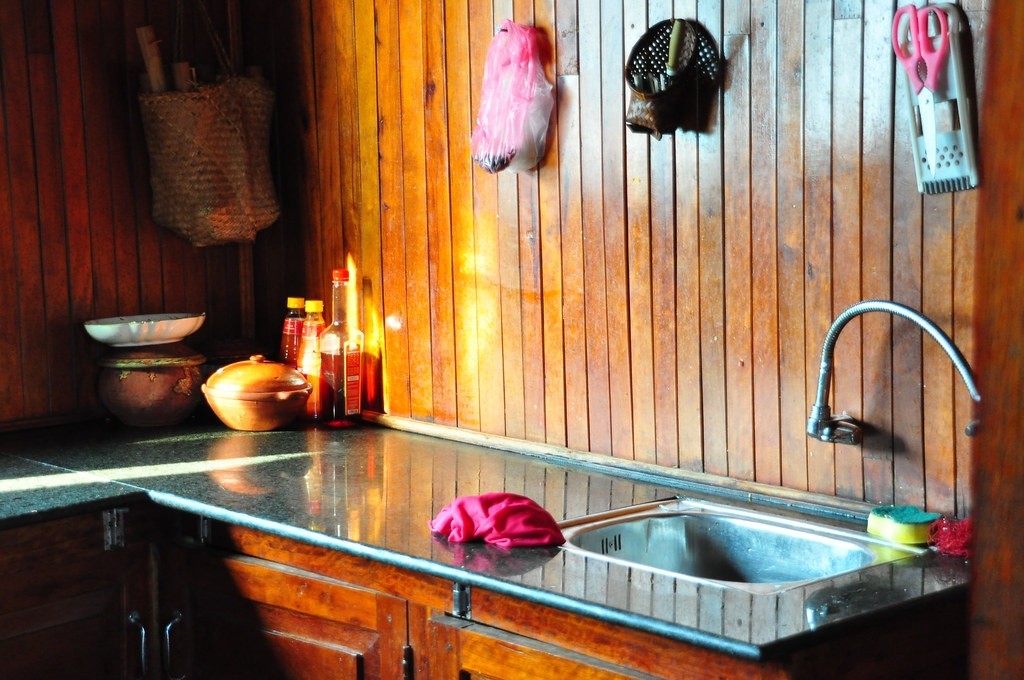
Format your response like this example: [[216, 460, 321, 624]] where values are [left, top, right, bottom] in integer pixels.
[[2, 502, 969, 680]]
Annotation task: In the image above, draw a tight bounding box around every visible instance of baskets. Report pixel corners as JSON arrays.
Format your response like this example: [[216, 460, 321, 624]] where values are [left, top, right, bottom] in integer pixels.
[[139, 0, 281, 247]]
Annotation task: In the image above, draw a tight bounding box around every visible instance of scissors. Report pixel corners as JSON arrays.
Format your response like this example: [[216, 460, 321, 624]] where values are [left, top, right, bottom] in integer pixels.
[[891, 5, 950, 180]]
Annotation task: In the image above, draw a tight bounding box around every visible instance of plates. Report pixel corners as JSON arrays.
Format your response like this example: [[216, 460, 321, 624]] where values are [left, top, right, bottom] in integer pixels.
[[84, 313, 206, 347]]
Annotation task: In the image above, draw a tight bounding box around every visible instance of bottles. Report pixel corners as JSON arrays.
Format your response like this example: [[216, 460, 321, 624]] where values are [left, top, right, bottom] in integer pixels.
[[319, 270, 364, 427], [296, 300, 326, 421], [279, 297, 305, 370]]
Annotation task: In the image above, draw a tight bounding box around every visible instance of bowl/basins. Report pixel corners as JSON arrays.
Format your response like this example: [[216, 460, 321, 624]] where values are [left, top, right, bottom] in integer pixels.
[[95, 343, 207, 428], [201, 355, 313, 432]]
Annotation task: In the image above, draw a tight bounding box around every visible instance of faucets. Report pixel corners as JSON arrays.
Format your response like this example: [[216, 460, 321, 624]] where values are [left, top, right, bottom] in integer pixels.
[[807, 302, 981, 445]]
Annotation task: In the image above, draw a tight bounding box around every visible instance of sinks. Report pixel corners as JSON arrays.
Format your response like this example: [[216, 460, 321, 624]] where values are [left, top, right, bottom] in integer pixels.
[[556, 493, 925, 596]]
